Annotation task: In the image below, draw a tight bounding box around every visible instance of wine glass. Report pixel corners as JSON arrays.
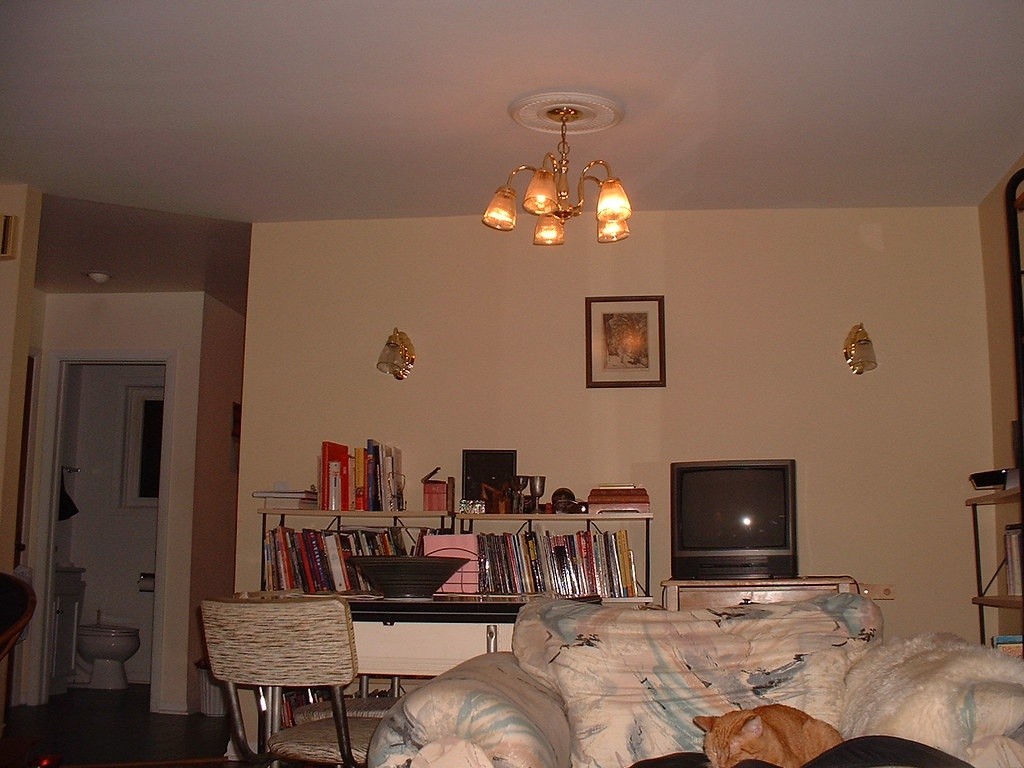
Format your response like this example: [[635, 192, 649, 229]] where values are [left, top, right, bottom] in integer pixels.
[[529, 475, 547, 514], [511, 475, 529, 513]]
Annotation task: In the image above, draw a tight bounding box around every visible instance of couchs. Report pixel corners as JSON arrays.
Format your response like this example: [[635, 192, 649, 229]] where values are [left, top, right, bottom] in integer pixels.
[[365, 594, 1023, 768]]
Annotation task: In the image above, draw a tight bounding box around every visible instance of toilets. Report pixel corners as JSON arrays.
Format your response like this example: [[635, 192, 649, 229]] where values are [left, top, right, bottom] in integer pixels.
[[65, 619, 141, 690]]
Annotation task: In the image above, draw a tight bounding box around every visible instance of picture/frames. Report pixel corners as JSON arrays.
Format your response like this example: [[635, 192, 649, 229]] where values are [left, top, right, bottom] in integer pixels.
[[585, 295, 665, 388]]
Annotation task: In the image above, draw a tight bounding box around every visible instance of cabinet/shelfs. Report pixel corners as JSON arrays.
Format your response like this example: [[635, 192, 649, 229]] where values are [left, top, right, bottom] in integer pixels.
[[257, 508, 653, 696], [661, 574, 860, 610], [966, 484, 1024, 658]]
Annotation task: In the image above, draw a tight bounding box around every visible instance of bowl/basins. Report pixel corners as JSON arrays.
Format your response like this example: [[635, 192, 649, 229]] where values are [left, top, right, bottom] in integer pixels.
[[347, 555, 472, 601]]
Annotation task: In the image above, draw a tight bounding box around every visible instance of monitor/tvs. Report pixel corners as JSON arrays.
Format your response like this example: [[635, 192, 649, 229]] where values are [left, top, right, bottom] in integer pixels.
[[669, 457, 797, 580]]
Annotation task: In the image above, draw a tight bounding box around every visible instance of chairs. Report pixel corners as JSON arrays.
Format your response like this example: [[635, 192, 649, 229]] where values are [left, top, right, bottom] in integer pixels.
[[200, 592, 382, 768]]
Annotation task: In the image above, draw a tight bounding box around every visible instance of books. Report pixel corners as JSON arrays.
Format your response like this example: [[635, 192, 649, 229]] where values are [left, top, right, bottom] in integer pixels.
[[989, 522, 1023, 661], [257, 681, 395, 738], [259, 440, 657, 614]]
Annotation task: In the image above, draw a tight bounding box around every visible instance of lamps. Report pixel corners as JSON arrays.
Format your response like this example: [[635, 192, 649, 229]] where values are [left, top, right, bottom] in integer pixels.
[[840, 322, 877, 376], [376, 325, 417, 380], [479, 108, 632, 243]]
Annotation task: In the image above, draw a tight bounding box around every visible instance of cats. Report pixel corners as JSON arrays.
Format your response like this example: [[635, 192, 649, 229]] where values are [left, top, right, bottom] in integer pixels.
[[693, 703, 844, 768]]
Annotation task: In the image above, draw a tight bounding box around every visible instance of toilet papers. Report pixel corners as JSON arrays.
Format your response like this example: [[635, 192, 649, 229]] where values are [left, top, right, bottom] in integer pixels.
[[137, 578, 155, 592]]
